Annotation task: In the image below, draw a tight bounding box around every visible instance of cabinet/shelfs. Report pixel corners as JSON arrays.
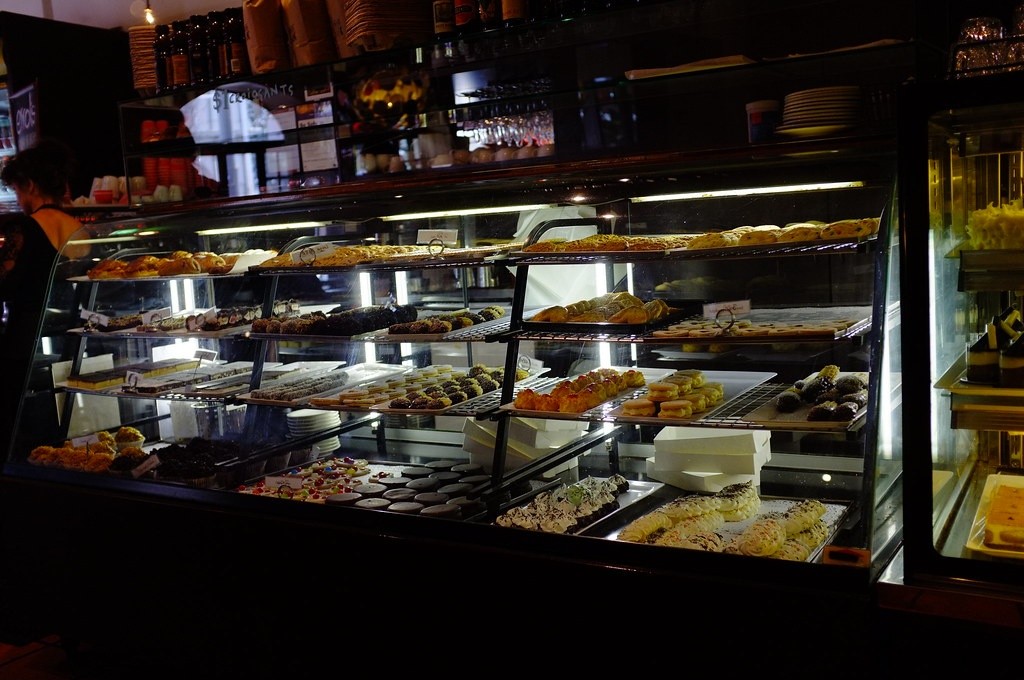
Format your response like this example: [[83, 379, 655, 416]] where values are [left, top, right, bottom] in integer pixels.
[[0, 143, 947, 597], [900, 36, 1024, 582], [116, 0, 899, 205]]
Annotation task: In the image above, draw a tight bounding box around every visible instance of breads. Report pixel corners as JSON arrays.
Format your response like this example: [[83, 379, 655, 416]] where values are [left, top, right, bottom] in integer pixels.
[[523, 233, 702, 250], [87, 251, 240, 276], [652, 319, 858, 335], [259, 245, 440, 268], [688, 217, 879, 249]]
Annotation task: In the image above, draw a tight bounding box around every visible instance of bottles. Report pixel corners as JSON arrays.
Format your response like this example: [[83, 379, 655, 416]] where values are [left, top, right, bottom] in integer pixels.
[[433, 0, 457, 42], [155, 22, 171, 93], [172, 22, 188, 89], [211, 10, 229, 78], [501, 0, 527, 29], [477, 0, 500, 32], [189, 13, 207, 86], [0, 107, 11, 148], [453, 0, 478, 35], [229, 9, 246, 74]]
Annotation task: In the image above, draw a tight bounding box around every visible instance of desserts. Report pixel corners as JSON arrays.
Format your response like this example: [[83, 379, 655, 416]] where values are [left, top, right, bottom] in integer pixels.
[[388, 365, 529, 410], [66, 359, 256, 395], [238, 457, 531, 521], [250, 365, 464, 407], [388, 305, 504, 334], [495, 472, 629, 536], [776, 364, 867, 421], [621, 370, 723, 419], [383, 241, 523, 264], [27, 426, 311, 491], [514, 367, 646, 413], [616, 481, 828, 561], [251, 304, 418, 336], [91, 298, 300, 332]]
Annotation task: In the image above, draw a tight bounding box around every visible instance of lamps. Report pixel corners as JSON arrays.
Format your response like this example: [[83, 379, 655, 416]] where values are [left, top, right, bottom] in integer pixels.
[[144, 0, 155, 24]]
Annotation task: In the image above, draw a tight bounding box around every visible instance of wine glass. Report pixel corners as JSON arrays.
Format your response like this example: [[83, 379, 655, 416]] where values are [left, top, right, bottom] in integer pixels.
[[464, 80, 556, 147]]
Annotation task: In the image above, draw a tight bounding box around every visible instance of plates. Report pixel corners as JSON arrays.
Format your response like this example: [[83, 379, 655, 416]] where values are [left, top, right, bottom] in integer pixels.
[[775, 85, 861, 136], [286, 408, 343, 459], [128, 25, 174, 94], [344, 0, 431, 51]]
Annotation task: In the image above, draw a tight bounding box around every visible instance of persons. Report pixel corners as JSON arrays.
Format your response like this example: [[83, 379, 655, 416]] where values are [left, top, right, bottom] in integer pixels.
[[0, 147, 101, 469]]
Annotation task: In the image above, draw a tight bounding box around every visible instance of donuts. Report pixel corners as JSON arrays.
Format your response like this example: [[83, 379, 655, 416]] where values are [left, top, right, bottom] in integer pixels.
[[529, 291, 670, 325]]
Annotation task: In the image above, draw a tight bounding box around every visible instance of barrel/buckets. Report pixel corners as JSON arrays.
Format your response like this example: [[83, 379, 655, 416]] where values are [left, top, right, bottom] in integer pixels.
[[745, 100, 780, 143]]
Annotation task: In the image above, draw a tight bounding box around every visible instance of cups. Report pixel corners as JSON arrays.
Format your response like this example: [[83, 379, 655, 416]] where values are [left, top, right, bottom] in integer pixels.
[[87, 174, 143, 202], [469, 148, 494, 163], [140, 120, 193, 201], [511, 147, 536, 159], [532, 146, 553, 157], [489, 147, 514, 161], [450, 149, 468, 165]]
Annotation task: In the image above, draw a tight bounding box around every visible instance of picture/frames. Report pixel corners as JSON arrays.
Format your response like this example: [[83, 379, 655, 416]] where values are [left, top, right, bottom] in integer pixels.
[[303, 80, 334, 101]]
